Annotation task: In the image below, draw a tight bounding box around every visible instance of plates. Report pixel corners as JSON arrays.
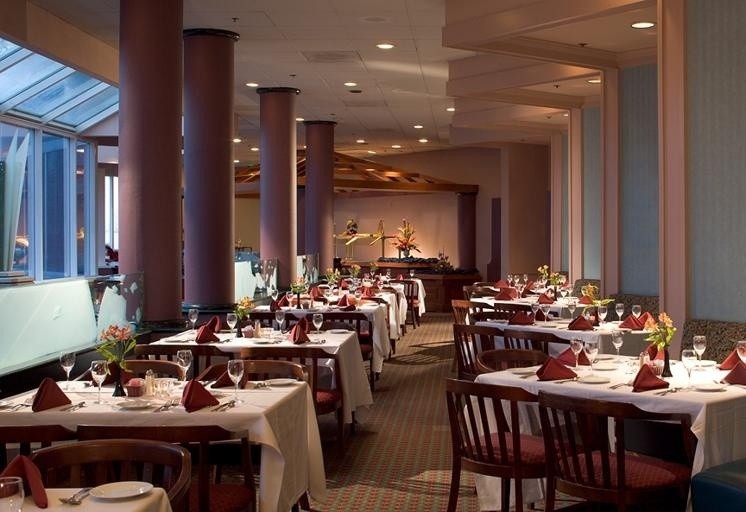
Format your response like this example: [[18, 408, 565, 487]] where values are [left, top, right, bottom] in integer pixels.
[[116, 400, 151, 409], [511, 354, 729, 392], [254, 339, 274, 344], [88, 481, 153, 501], [488, 319, 625, 331], [264, 378, 296, 387], [328, 328, 349, 334]]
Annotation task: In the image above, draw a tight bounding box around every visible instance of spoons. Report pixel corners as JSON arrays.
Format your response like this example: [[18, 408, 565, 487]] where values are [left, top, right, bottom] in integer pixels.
[[69, 493, 89, 504]]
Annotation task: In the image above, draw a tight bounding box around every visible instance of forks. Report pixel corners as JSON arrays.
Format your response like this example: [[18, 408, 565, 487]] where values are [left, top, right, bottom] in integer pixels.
[[610, 377, 635, 389], [161, 398, 180, 411], [59, 487, 91, 504], [153, 400, 172, 413]]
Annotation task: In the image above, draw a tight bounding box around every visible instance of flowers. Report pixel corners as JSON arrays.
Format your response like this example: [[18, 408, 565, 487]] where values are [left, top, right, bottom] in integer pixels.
[[391, 219, 421, 254]]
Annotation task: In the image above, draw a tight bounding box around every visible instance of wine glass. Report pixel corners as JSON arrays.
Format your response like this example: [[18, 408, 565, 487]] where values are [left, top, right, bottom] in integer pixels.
[[0, 477, 25, 512], [176, 349, 194, 389], [91, 360, 109, 405], [275, 310, 285, 334], [682, 350, 696, 380], [569, 335, 598, 376], [504, 273, 547, 300], [377, 281, 384, 296], [226, 314, 237, 340], [286, 292, 294, 310], [228, 360, 245, 404], [271, 290, 278, 301], [60, 351, 76, 391], [313, 314, 324, 341], [560, 282, 575, 304], [528, 300, 642, 329], [610, 331, 624, 364], [693, 335, 706, 366], [189, 309, 199, 334], [363, 268, 414, 285], [355, 291, 362, 311]]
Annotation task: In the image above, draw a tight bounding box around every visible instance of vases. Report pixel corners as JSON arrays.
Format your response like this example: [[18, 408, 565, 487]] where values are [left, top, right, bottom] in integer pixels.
[[404, 250, 409, 258]]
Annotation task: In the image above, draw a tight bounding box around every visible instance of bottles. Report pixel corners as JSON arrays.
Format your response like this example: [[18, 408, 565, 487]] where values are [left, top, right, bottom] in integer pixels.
[[145, 369, 156, 396]]
